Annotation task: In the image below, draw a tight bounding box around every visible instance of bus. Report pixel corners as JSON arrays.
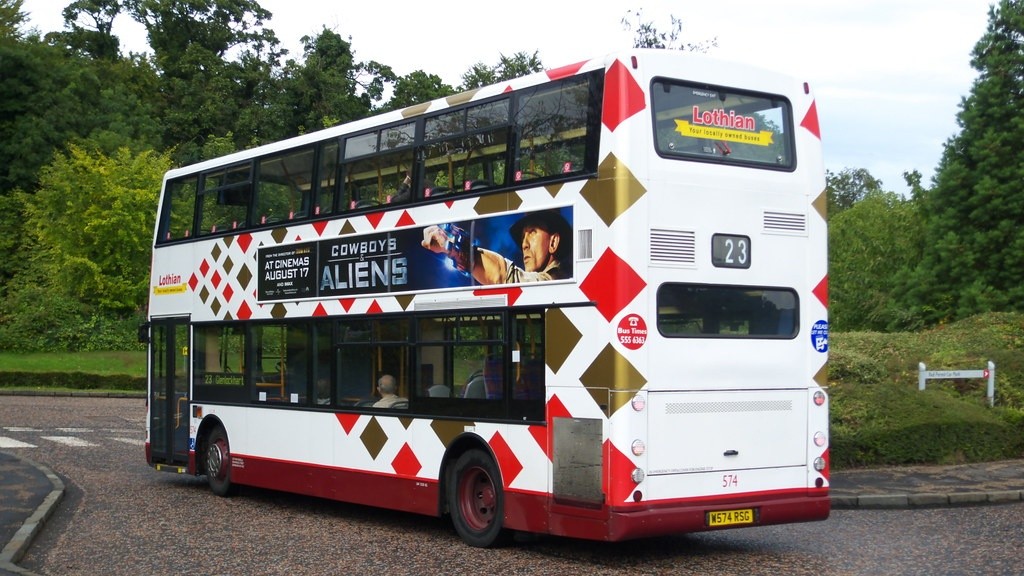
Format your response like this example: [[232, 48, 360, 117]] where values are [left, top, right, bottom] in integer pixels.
[[137, 49, 832, 550]]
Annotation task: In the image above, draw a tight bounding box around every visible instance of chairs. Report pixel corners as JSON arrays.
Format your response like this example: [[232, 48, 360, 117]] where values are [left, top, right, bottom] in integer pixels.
[[167, 162, 582, 241], [424, 369, 497, 399], [354, 400, 409, 408]]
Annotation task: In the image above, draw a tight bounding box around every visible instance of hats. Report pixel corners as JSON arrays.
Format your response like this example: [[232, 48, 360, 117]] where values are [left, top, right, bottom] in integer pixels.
[[509, 208, 572, 261]]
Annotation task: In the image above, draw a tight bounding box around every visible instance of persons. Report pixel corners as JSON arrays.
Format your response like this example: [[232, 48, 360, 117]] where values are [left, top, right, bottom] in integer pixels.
[[421, 210, 572, 284], [390, 167, 438, 202], [371, 375, 408, 408]]
